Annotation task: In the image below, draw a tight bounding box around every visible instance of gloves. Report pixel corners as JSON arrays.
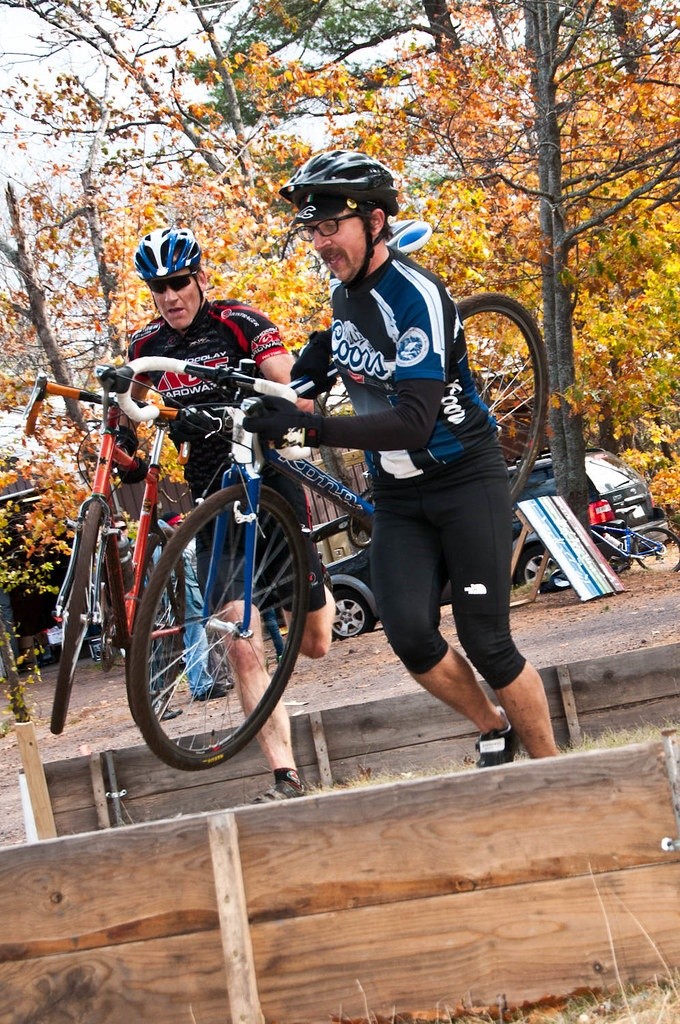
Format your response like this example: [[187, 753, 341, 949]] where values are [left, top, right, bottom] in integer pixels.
[[166, 409, 231, 445], [289, 332, 339, 398], [117, 426, 147, 484], [242, 397, 324, 454]]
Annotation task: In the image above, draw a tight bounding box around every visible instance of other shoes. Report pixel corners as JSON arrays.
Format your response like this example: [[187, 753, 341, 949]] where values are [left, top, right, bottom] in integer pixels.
[[156, 706, 184, 721], [248, 780, 306, 803], [216, 678, 235, 690], [476, 712, 520, 768], [201, 686, 228, 701]]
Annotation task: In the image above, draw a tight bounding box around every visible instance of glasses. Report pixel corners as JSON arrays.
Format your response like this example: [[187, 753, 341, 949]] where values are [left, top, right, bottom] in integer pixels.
[[292, 213, 359, 242], [146, 269, 199, 293]]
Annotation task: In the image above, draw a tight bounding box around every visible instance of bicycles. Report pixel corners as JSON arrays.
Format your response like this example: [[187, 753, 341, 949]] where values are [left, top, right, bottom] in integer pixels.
[[19, 371, 310, 737], [587, 506, 680, 576], [83, 291, 553, 770]]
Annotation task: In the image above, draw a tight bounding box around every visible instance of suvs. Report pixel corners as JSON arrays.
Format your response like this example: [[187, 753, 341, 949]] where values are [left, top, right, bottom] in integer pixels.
[[316, 446, 655, 644]]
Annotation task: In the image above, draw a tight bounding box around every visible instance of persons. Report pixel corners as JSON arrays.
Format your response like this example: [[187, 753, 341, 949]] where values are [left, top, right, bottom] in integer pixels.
[[183, 536, 235, 691], [244, 142, 568, 771], [138, 506, 224, 722], [94, 224, 345, 807]]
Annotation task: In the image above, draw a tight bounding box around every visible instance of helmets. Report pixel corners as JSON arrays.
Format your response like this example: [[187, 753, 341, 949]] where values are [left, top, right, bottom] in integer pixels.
[[134, 226, 201, 281], [279, 150, 400, 217]]
